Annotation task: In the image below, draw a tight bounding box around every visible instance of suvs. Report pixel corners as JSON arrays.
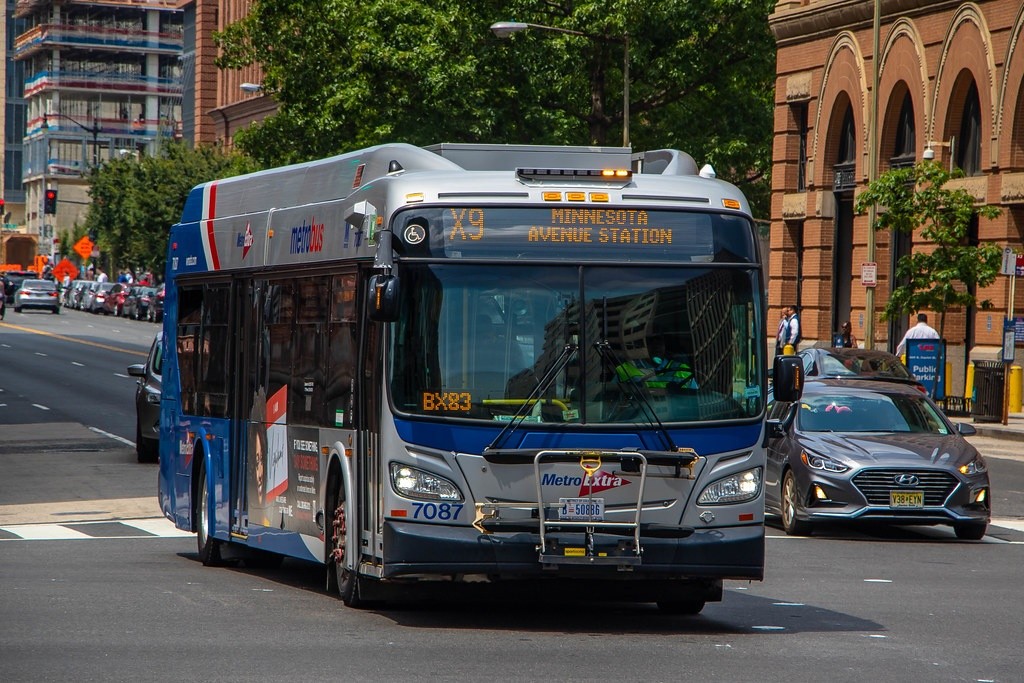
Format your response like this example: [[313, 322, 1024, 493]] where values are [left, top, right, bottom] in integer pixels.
[[2, 271, 40, 303]]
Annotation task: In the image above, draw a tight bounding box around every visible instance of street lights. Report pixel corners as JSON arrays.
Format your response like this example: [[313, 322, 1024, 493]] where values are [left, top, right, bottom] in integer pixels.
[[490, 22, 628, 150], [41, 112, 99, 216]]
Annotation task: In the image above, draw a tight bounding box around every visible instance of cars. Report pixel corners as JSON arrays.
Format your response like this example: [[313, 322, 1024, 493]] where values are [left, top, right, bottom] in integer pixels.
[[763, 380, 991, 539], [129, 286, 159, 320], [121, 287, 142, 318], [768, 347, 926, 406], [127, 332, 164, 463], [64, 281, 86, 307], [69, 281, 98, 308], [14, 279, 60, 314], [90, 283, 116, 314], [80, 283, 101, 311], [146, 283, 165, 322], [103, 283, 141, 317]]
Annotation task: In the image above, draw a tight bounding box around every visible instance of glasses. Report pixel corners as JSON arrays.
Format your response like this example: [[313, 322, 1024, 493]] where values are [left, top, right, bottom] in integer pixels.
[[842, 326, 847, 329]]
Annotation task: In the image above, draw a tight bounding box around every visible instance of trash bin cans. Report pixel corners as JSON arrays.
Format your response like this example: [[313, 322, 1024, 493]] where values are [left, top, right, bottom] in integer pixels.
[[971, 359, 1006, 422]]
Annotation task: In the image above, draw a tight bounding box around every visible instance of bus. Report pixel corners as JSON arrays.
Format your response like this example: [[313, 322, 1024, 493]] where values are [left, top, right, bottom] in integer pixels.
[[157, 144, 805, 616]]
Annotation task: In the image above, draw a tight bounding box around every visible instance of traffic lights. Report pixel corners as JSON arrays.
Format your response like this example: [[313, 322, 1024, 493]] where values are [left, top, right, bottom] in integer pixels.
[[0, 198, 4, 216], [44, 189, 58, 214]]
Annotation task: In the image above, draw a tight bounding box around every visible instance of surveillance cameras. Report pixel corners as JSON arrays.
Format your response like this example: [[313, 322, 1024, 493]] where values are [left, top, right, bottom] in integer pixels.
[[923, 151, 935, 161]]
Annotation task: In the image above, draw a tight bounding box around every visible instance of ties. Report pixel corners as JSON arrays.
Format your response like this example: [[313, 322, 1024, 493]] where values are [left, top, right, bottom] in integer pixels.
[[777, 322, 784, 340]]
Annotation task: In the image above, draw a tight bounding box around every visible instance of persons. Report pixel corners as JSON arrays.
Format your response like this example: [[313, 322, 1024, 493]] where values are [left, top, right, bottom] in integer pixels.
[[611, 328, 700, 390], [246, 423, 271, 527], [832, 322, 858, 349], [769, 305, 802, 386], [406, 306, 523, 397], [896, 313, 940, 359], [41, 260, 166, 286]]
